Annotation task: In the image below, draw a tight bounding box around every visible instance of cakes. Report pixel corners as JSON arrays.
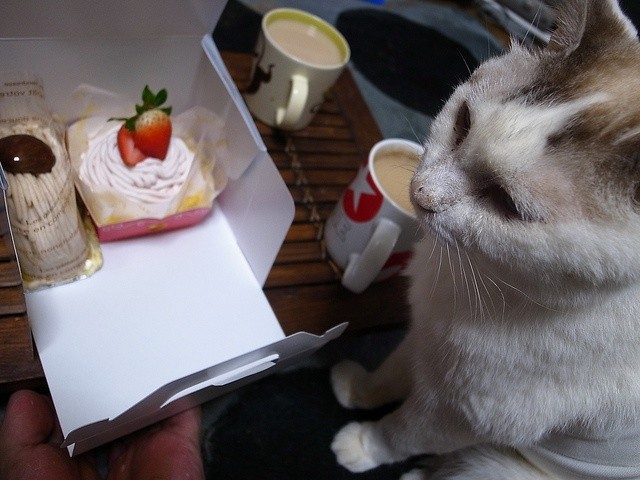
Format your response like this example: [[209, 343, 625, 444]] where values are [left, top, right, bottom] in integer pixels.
[[68, 84, 199, 217]]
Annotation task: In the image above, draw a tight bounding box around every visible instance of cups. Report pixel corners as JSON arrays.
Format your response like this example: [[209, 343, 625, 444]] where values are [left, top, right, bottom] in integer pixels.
[[244, 8, 351, 132], [323, 138, 429, 295]]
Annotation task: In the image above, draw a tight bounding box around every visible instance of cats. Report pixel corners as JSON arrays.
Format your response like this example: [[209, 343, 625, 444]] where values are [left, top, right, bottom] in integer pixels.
[[321, 0, 640, 480]]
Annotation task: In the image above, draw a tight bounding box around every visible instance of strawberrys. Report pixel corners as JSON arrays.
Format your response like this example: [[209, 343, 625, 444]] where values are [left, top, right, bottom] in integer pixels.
[[126, 86, 171, 161], [107, 117, 147, 166]]
